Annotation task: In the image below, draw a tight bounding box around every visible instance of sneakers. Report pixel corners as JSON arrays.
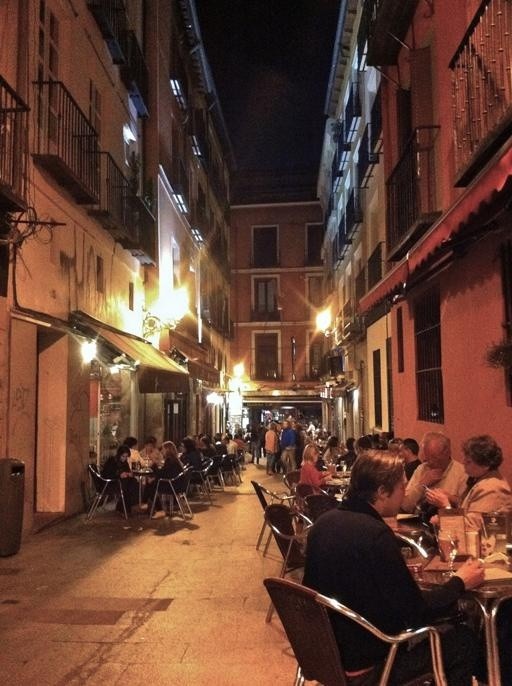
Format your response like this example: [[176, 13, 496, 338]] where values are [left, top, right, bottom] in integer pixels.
[[151, 510, 166, 520], [140, 503, 148, 510]]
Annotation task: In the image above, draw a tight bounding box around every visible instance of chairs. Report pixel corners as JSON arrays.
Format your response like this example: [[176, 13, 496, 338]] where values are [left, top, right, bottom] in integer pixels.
[[262, 502, 313, 627], [261, 575, 448, 685]]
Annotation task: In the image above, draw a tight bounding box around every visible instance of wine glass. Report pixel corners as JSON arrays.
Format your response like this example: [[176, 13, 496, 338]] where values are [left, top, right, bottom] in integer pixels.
[[339, 482, 346, 495], [437, 528, 460, 578], [340, 460, 347, 477]]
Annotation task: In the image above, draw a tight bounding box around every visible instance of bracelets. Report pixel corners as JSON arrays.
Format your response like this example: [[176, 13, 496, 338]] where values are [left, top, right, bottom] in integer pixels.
[[443, 503, 452, 510]]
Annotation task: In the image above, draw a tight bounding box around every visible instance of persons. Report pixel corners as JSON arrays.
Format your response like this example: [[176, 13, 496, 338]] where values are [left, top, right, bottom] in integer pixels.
[[424, 436, 512, 533], [402, 431, 468, 509], [299, 449, 485, 686], [303, 421, 422, 495], [246, 415, 305, 482], [102, 432, 245, 518]]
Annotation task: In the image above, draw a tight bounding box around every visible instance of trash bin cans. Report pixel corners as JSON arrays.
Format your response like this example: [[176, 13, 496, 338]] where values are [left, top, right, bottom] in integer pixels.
[[1, 458, 26, 559]]
